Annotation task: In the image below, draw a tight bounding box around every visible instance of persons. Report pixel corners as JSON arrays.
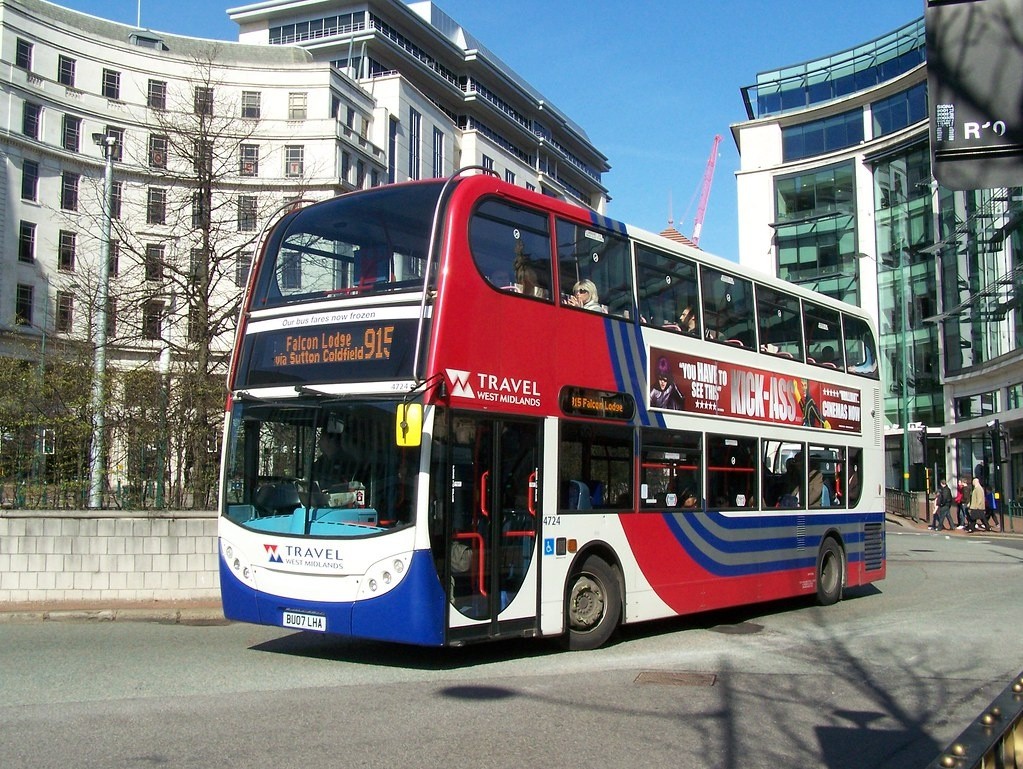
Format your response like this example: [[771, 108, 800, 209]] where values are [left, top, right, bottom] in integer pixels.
[[299, 425, 359, 494], [928, 478, 1001, 533], [645, 453, 860, 509], [498, 264, 844, 368]]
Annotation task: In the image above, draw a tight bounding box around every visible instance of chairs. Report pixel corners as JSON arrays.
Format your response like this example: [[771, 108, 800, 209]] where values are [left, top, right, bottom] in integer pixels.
[[661, 325, 839, 368], [654, 492, 684, 507], [562, 480, 633, 509], [776, 494, 798, 507], [821, 485, 831, 507]]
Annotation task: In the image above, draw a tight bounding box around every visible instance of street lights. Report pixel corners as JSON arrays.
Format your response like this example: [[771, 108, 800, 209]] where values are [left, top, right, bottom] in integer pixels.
[[89, 131, 120, 509], [854, 239, 912, 517]]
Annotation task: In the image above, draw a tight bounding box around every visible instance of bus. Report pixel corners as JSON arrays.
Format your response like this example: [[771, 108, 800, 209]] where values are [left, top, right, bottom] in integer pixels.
[[216, 164, 889, 653]]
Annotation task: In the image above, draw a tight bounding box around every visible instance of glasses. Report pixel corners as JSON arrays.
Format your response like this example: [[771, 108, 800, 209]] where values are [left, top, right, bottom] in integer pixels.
[[573, 288, 588, 295]]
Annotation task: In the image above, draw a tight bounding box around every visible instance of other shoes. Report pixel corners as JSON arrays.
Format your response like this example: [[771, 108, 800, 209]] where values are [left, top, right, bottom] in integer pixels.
[[981, 524, 985, 528], [975, 524, 978, 528], [967, 529, 973, 533], [956, 525, 965, 529], [935, 527, 939, 531], [984, 528, 991, 531], [949, 528, 955, 530], [928, 525, 934, 529]]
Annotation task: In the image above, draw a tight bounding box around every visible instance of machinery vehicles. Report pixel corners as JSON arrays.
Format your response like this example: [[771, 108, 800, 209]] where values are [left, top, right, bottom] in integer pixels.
[[666, 133, 724, 246]]
